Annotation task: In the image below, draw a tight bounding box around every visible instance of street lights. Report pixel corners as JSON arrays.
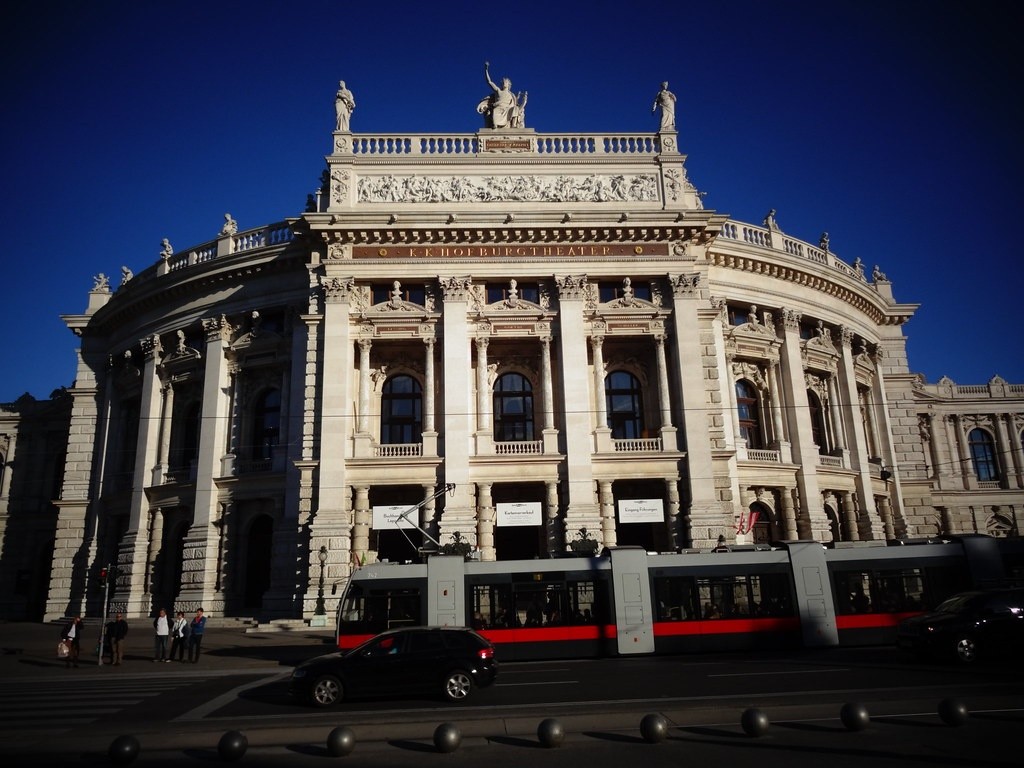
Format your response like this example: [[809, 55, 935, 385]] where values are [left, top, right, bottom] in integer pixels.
[[315, 547, 328, 616]]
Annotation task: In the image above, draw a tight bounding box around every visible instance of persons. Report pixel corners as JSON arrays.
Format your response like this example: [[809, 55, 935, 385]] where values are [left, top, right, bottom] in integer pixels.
[[764, 209, 777, 229], [656, 597, 793, 621], [652, 81, 678, 130], [392, 281, 403, 297], [92, 213, 238, 292], [853, 257, 864, 272], [154, 607, 206, 664], [111, 613, 128, 665], [358, 173, 660, 203], [62, 617, 84, 669], [819, 232, 830, 250], [478, 608, 595, 628], [177, 330, 186, 349], [335, 80, 355, 131], [623, 277, 634, 297], [508, 279, 518, 299], [748, 305, 760, 324], [326, 275, 699, 311], [484, 62, 528, 129]]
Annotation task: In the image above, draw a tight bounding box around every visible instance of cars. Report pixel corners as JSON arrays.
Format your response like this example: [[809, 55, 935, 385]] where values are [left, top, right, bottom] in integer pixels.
[[893, 586, 1024, 660], [288, 626, 499, 708]]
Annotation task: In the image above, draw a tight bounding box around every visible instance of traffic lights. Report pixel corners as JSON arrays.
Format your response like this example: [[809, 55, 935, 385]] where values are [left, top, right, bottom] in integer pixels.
[[100, 568, 107, 580], [110, 566, 117, 580]]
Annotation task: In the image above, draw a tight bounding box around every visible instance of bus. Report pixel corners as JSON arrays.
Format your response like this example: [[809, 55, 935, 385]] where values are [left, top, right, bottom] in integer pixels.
[[334, 534, 1024, 659]]
